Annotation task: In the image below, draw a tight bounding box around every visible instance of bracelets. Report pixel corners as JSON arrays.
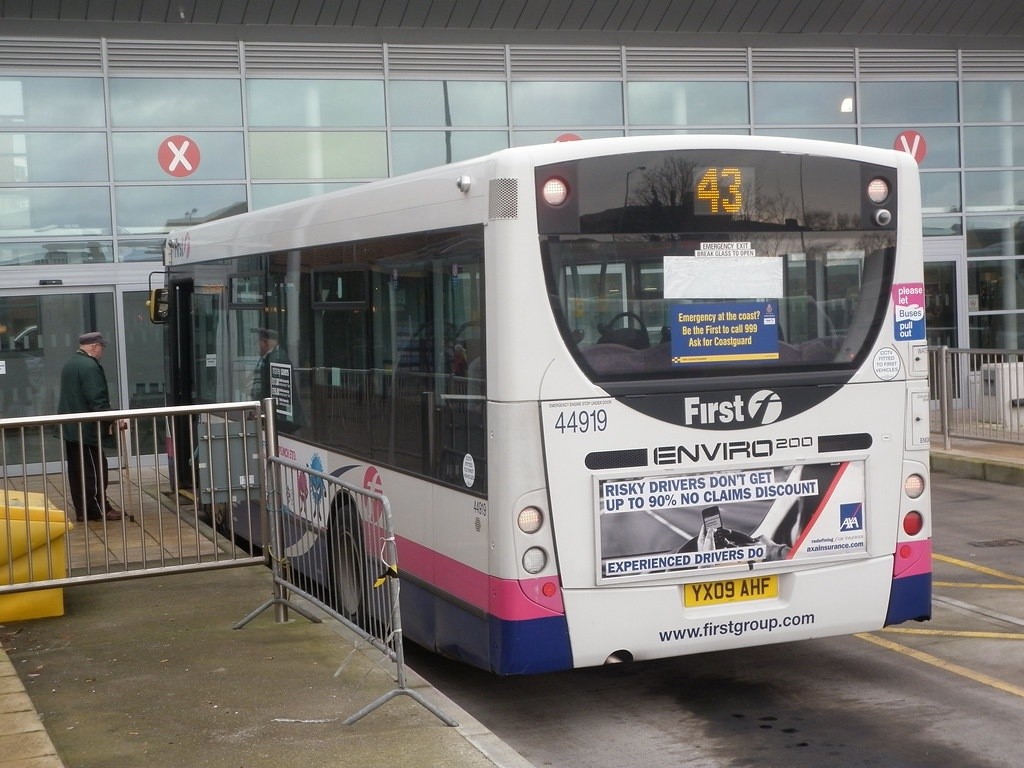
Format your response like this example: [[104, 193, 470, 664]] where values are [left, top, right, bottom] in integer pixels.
[[777, 543, 788, 558]]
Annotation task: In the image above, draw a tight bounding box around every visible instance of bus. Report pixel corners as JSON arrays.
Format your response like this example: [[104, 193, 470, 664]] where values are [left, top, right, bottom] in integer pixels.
[[143, 134, 935, 677]]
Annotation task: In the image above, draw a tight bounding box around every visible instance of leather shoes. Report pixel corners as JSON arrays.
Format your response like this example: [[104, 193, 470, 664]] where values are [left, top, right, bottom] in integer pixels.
[[101, 509, 127, 520], [76, 510, 102, 521]]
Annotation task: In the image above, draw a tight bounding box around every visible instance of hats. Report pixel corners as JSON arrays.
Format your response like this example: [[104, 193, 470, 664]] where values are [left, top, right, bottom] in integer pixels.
[[260, 329, 279, 339], [79, 332, 110, 345]]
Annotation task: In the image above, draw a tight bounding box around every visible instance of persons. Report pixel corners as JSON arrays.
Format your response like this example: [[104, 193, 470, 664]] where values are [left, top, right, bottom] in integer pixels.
[[58, 331, 127, 522], [253, 330, 306, 436], [698, 489, 822, 569]]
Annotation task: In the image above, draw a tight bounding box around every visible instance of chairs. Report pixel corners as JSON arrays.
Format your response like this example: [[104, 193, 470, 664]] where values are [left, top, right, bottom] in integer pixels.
[[395, 311, 671, 410]]
